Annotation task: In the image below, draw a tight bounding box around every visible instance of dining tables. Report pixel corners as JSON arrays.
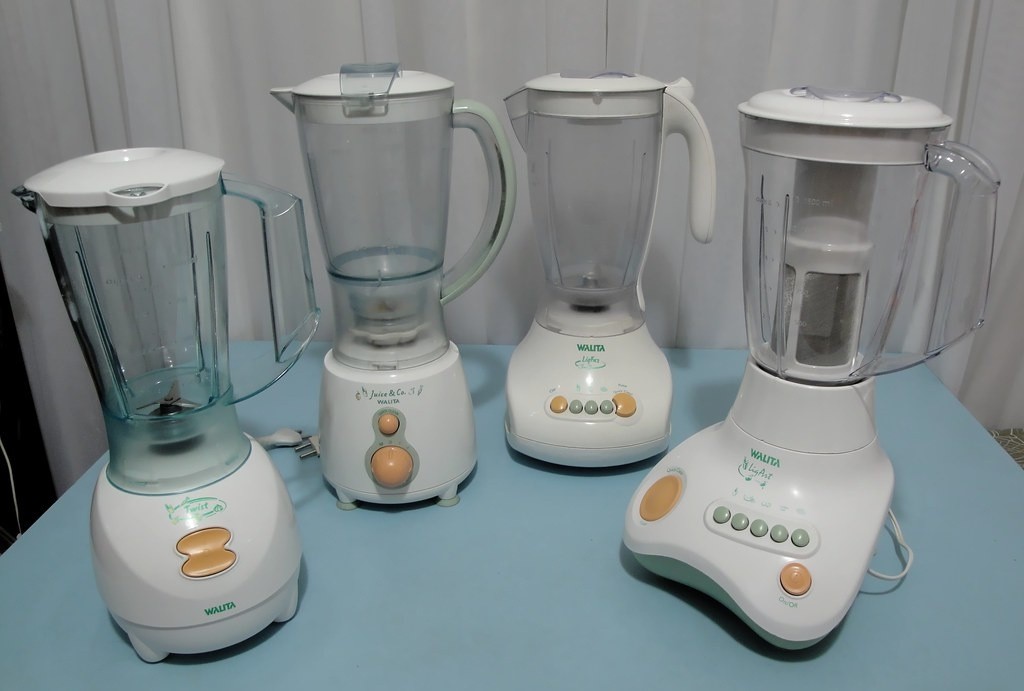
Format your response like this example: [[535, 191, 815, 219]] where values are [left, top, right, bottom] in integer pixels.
[[1, 340, 1024, 691]]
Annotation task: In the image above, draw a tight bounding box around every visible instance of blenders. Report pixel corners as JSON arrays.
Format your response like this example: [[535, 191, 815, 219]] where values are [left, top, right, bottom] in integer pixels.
[[270, 58, 517, 515], [501, 68, 720, 475], [617, 80, 1004, 656], [5, 145, 322, 664]]
